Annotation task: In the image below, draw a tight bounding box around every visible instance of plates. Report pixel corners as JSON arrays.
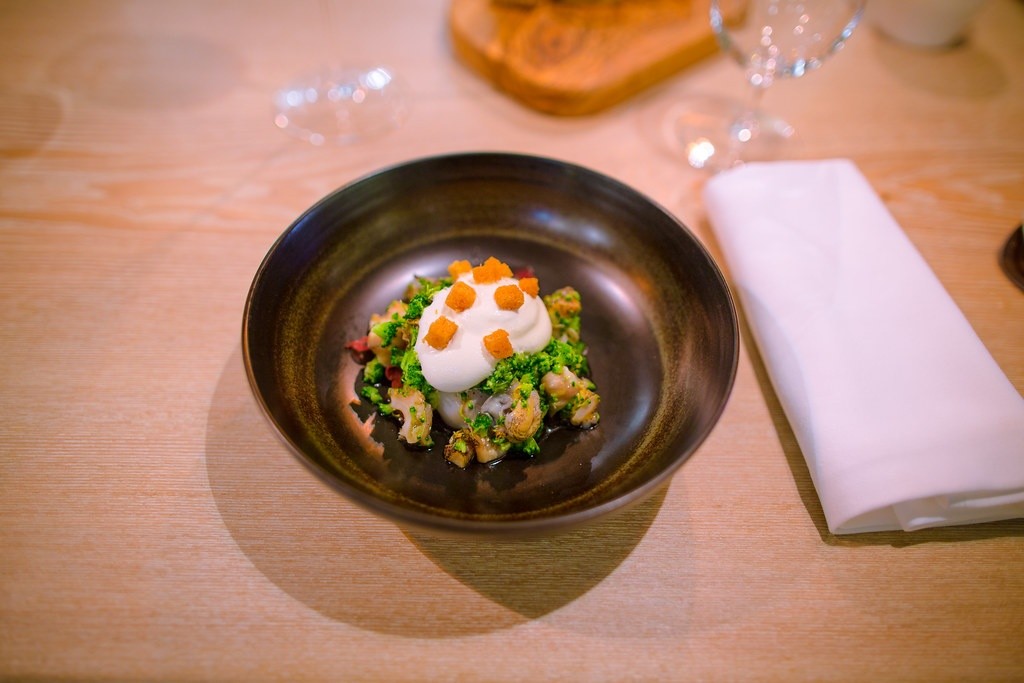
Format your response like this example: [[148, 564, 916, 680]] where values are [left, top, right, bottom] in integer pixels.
[[241, 152, 739, 526]]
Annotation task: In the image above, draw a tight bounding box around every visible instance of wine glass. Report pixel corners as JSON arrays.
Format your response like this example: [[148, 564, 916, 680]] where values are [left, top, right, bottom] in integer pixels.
[[669, 0, 866, 171]]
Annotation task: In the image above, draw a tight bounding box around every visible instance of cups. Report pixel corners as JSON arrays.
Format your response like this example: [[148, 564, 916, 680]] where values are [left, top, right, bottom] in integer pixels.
[[866, 0, 982, 48]]
